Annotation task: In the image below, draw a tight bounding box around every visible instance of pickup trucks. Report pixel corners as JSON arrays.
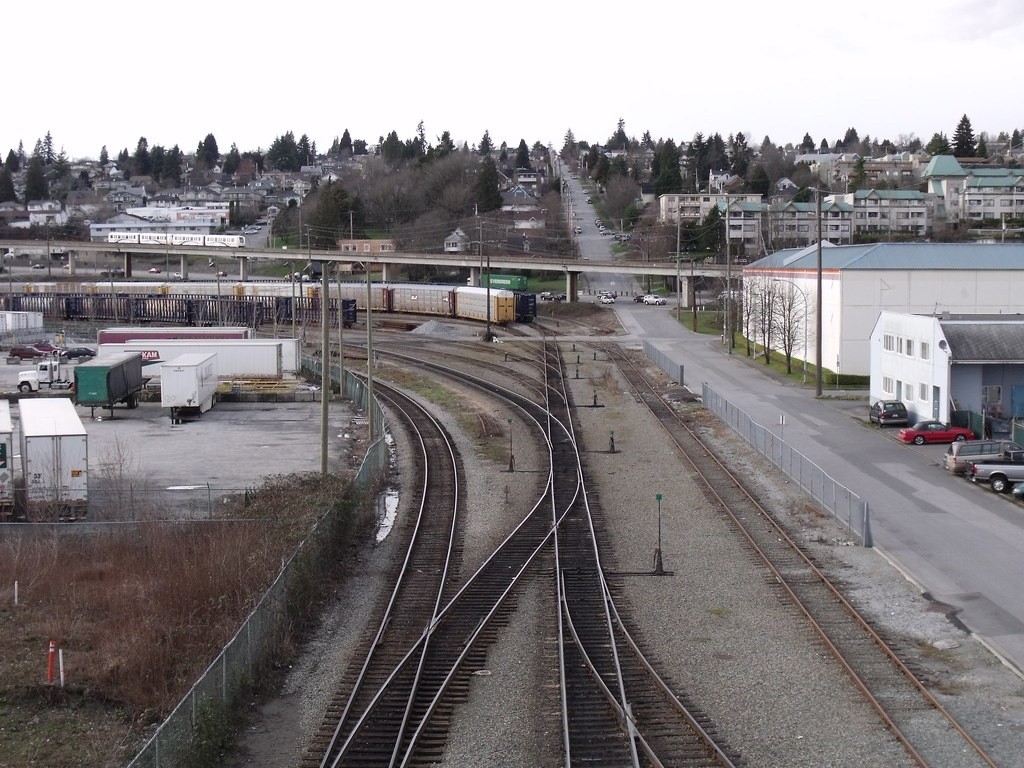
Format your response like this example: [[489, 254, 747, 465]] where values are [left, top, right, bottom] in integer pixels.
[[966, 450, 1024, 493]]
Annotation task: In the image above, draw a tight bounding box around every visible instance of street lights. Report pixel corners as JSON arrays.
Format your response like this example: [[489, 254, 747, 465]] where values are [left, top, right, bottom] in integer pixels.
[[773, 278, 810, 383]]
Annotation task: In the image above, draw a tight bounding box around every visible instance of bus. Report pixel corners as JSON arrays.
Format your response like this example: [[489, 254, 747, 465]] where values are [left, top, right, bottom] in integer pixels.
[[108, 230, 246, 248]]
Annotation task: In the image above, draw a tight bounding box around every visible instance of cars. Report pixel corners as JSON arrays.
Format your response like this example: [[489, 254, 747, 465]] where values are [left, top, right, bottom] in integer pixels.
[[586, 196, 592, 204], [173, 271, 181, 278], [634, 295, 644, 303], [243, 219, 268, 235], [558, 292, 579, 301], [33, 263, 45, 269], [215, 271, 228, 276], [63, 264, 69, 269], [897, 420, 975, 445], [582, 188, 588, 194], [148, 267, 161, 273], [594, 216, 631, 242], [111, 266, 124, 274], [8, 338, 96, 360], [1011, 482, 1024, 500], [596, 291, 617, 304], [575, 225, 582, 234], [869, 399, 909, 428]]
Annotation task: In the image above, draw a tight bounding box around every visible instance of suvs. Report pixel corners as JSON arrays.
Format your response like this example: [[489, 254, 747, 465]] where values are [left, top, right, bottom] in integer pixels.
[[540, 291, 558, 302], [643, 294, 668, 306], [943, 439, 1024, 474]]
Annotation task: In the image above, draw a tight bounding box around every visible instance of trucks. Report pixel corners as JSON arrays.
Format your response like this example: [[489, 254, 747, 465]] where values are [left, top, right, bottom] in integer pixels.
[[16, 361, 74, 393], [466, 273, 528, 292]]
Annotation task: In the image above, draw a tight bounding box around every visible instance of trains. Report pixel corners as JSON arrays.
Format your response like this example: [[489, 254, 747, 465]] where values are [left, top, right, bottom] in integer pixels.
[[0, 277, 516, 326], [513, 292, 537, 323]]
